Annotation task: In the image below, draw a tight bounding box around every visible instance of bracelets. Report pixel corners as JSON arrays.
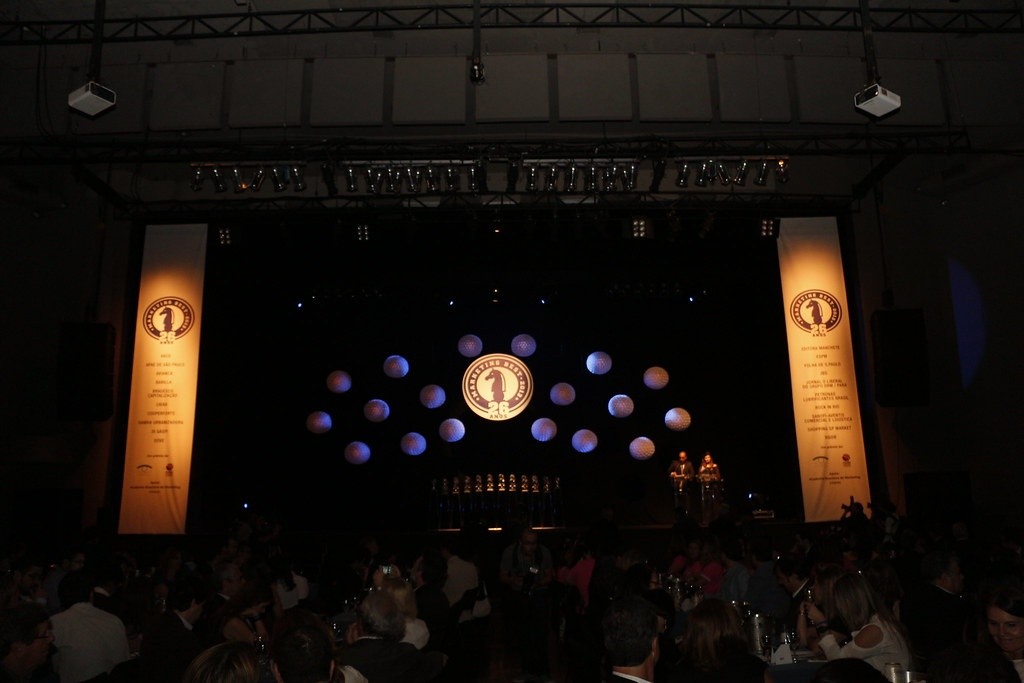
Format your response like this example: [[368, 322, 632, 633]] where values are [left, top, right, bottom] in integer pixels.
[[799, 610, 806, 615], [817, 620, 828, 625]]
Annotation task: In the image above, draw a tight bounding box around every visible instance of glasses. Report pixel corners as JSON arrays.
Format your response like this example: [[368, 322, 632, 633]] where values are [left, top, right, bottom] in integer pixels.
[[31, 628, 49, 642]]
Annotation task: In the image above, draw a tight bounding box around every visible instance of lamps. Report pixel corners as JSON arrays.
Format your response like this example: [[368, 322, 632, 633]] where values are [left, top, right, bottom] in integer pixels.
[[344, 165, 361, 192], [289, 164, 308, 192], [564, 161, 579, 192], [506, 162, 519, 192], [526, 162, 539, 190], [268, 165, 288, 193], [544, 163, 559, 192], [363, 165, 423, 193], [424, 165, 438, 192], [320, 161, 339, 196], [230, 167, 248, 194], [466, 164, 480, 190], [208, 166, 229, 194], [190, 166, 205, 192], [445, 164, 458, 192], [471, 62, 486, 85], [246, 166, 267, 192], [584, 161, 599, 191], [602, 157, 790, 192]]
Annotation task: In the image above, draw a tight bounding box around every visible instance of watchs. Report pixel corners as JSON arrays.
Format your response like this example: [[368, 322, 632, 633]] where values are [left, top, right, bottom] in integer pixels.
[[816, 627, 830, 633]]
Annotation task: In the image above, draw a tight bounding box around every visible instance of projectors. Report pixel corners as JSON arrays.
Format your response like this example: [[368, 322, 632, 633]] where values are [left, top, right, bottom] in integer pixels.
[[854, 84, 901, 117], [68, 81, 116, 116]]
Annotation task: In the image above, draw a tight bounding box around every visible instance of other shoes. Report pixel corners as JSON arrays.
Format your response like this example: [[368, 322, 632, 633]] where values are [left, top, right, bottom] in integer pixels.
[[512, 673, 540, 683], [541, 674, 555, 683]]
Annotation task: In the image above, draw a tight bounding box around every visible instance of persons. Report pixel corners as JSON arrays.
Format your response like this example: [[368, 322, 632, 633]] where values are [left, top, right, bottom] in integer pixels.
[[603, 602, 660, 683], [982, 587, 1024, 660], [670, 597, 774, 683], [925, 641, 1023, 683], [666, 451, 693, 526], [696, 453, 721, 525], [0, 503, 1024, 683]]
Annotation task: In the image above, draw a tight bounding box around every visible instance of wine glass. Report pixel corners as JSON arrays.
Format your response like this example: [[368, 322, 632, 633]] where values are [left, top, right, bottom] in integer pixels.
[[760, 636, 771, 658], [790, 632, 800, 664], [328, 593, 360, 639], [805, 588, 817, 624], [255, 634, 270, 679]]
